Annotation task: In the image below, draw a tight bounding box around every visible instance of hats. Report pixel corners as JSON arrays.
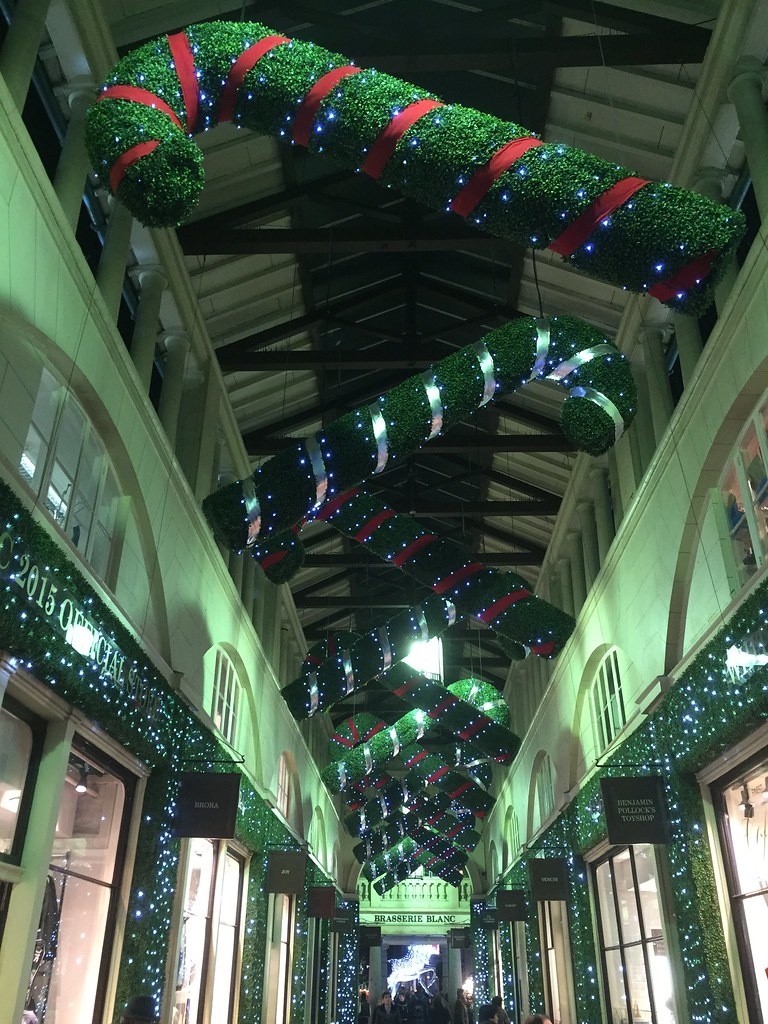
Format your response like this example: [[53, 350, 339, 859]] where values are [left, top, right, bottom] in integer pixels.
[[118, 997, 159, 1020]]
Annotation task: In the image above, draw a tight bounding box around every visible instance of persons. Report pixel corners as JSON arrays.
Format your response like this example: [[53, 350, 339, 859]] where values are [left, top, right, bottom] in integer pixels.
[[121, 996, 161, 1024], [371, 987, 550, 1024]]
